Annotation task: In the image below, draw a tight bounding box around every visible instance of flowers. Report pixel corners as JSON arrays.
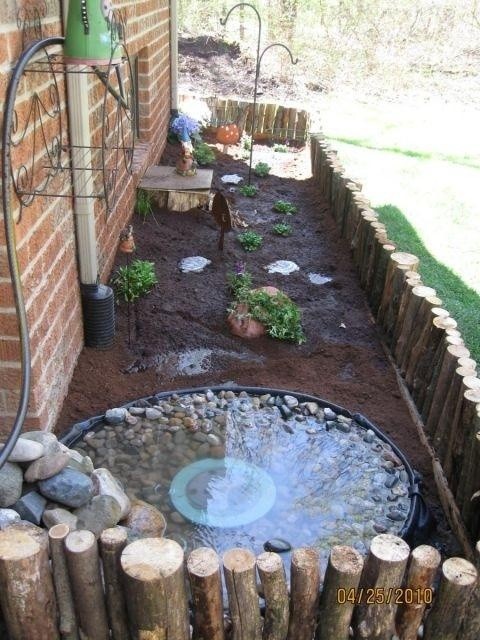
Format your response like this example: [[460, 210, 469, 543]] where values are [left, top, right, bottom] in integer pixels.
[[171, 113, 199, 148]]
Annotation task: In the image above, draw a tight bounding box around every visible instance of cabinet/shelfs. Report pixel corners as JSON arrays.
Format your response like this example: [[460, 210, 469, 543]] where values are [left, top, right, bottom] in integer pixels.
[[11, 31, 142, 222]]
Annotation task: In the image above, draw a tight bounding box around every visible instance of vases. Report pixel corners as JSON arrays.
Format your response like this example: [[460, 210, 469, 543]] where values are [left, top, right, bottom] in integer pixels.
[[176, 154, 198, 175]]
